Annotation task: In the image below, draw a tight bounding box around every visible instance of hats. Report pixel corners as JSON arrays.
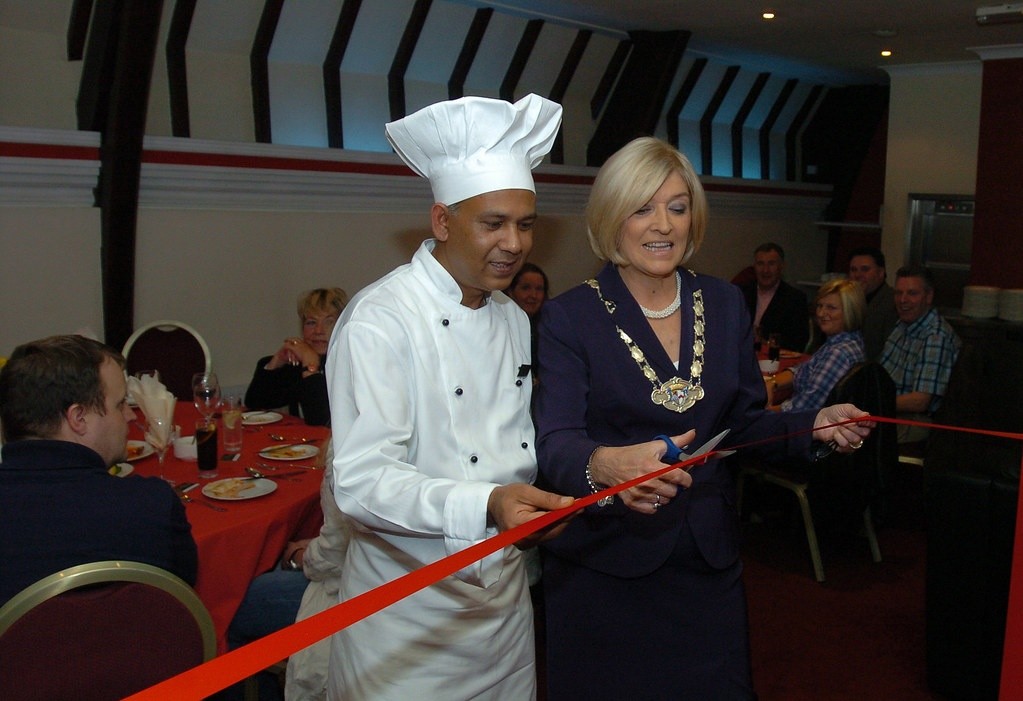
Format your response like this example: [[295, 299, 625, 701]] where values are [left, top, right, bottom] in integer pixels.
[[383, 93, 564, 208]]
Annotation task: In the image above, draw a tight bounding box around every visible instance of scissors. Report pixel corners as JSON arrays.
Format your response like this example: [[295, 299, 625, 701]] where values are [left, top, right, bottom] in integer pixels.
[[652, 428, 737, 474]]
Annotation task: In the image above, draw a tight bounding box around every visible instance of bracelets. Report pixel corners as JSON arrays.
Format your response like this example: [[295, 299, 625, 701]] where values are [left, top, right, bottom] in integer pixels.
[[586, 446, 614, 507], [770, 380, 778, 388]]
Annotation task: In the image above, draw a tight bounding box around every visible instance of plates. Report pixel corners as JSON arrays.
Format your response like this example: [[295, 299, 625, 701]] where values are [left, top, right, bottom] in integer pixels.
[[125, 439, 155, 462], [238, 411, 284, 425], [202, 476, 279, 500], [960, 285, 1023, 322], [258, 445, 320, 460], [778, 351, 802, 358]]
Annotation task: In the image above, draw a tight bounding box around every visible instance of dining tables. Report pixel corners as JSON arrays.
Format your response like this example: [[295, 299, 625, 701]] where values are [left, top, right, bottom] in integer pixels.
[[756, 350, 811, 408], [106, 401, 333, 659]]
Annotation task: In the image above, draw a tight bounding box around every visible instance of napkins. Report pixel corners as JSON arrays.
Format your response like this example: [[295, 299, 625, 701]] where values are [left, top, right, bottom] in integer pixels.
[[126, 368, 178, 450]]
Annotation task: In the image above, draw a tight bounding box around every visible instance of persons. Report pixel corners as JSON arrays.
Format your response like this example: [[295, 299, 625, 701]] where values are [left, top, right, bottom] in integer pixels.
[[243, 288, 348, 427], [505, 264, 551, 314], [223, 439, 350, 701], [847, 246, 896, 357], [0, 336, 199, 596], [535, 137, 875, 701], [766, 278, 866, 411], [740, 243, 809, 353], [326, 91, 585, 701], [874, 265, 963, 443]]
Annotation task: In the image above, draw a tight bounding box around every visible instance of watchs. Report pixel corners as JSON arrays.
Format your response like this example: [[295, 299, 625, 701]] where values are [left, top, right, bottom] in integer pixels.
[[286, 548, 303, 570], [176, 491, 226, 512], [302, 362, 322, 371]]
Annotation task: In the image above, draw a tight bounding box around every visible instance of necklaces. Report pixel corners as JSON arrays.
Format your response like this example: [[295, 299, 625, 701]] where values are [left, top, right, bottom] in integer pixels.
[[584, 270, 706, 413], [640, 272, 681, 318]]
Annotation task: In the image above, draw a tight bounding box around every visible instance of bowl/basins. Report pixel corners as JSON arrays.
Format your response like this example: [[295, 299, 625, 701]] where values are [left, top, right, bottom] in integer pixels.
[[109, 462, 134, 478]]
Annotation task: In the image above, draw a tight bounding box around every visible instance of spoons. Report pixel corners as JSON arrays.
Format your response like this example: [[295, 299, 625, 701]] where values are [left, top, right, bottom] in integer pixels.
[[269, 433, 314, 441], [181, 494, 226, 513], [245, 466, 305, 481]]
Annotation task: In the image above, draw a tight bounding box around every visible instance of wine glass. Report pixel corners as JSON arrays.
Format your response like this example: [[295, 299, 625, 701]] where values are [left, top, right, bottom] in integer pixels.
[[192, 384, 222, 426], [191, 372, 218, 403]]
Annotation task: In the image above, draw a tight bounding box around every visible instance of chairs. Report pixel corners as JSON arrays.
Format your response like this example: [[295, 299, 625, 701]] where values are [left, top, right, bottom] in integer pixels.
[[0, 560, 217, 701], [121, 319, 212, 401], [734, 340, 977, 583]]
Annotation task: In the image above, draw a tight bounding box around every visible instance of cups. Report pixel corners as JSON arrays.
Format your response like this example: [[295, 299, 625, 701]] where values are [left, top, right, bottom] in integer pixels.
[[195, 418, 219, 470], [767, 338, 780, 361], [172, 435, 199, 461], [220, 395, 244, 451], [757, 360, 780, 374]]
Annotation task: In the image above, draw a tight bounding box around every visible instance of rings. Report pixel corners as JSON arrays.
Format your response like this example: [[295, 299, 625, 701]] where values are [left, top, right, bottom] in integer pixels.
[[849, 440, 863, 449], [653, 495, 662, 509], [294, 341, 297, 344]]
